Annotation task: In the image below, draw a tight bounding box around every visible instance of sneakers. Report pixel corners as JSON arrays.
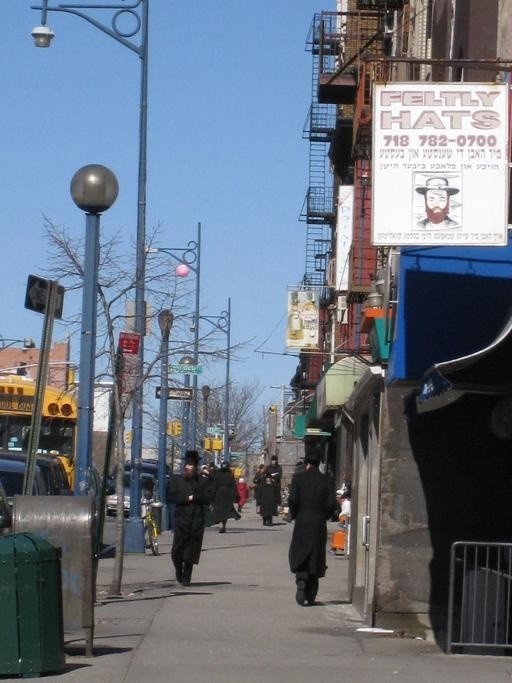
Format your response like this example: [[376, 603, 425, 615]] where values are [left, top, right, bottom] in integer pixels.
[[294, 578, 317, 607], [175, 567, 193, 585]]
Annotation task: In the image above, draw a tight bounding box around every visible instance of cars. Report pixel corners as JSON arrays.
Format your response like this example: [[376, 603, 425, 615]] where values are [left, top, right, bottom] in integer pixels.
[[106, 460, 171, 516], [0, 449, 68, 536]]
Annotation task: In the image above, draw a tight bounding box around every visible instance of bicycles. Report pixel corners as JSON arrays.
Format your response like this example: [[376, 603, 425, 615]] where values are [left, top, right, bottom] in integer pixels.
[[140, 496, 160, 556]]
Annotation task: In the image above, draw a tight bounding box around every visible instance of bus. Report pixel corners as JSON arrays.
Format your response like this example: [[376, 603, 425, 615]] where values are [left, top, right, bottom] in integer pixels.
[[1, 374, 79, 495]]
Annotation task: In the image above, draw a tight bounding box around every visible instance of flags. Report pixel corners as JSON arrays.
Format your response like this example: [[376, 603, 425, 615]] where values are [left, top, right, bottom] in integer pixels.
[[287, 289, 321, 350]]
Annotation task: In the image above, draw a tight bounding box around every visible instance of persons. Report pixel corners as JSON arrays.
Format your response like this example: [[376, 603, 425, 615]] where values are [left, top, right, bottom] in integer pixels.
[[165, 449, 212, 588], [198, 453, 306, 534], [413, 176, 462, 233], [286, 453, 338, 605]]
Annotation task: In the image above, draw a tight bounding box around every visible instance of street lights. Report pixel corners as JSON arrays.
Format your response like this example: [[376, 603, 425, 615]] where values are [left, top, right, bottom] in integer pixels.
[[70, 165, 118, 498], [31, 2, 145, 554], [203, 386, 211, 469], [192, 299, 232, 478], [157, 309, 175, 532], [177, 355, 200, 480], [145, 223, 201, 462]]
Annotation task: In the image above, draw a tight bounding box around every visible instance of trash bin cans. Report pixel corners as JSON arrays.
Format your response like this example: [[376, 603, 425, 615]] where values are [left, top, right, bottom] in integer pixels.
[[0, 533, 65, 679], [11, 494, 99, 658]]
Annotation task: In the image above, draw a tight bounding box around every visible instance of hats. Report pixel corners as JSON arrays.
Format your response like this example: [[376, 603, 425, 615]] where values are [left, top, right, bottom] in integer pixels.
[[299, 445, 327, 461], [179, 449, 203, 461]]
